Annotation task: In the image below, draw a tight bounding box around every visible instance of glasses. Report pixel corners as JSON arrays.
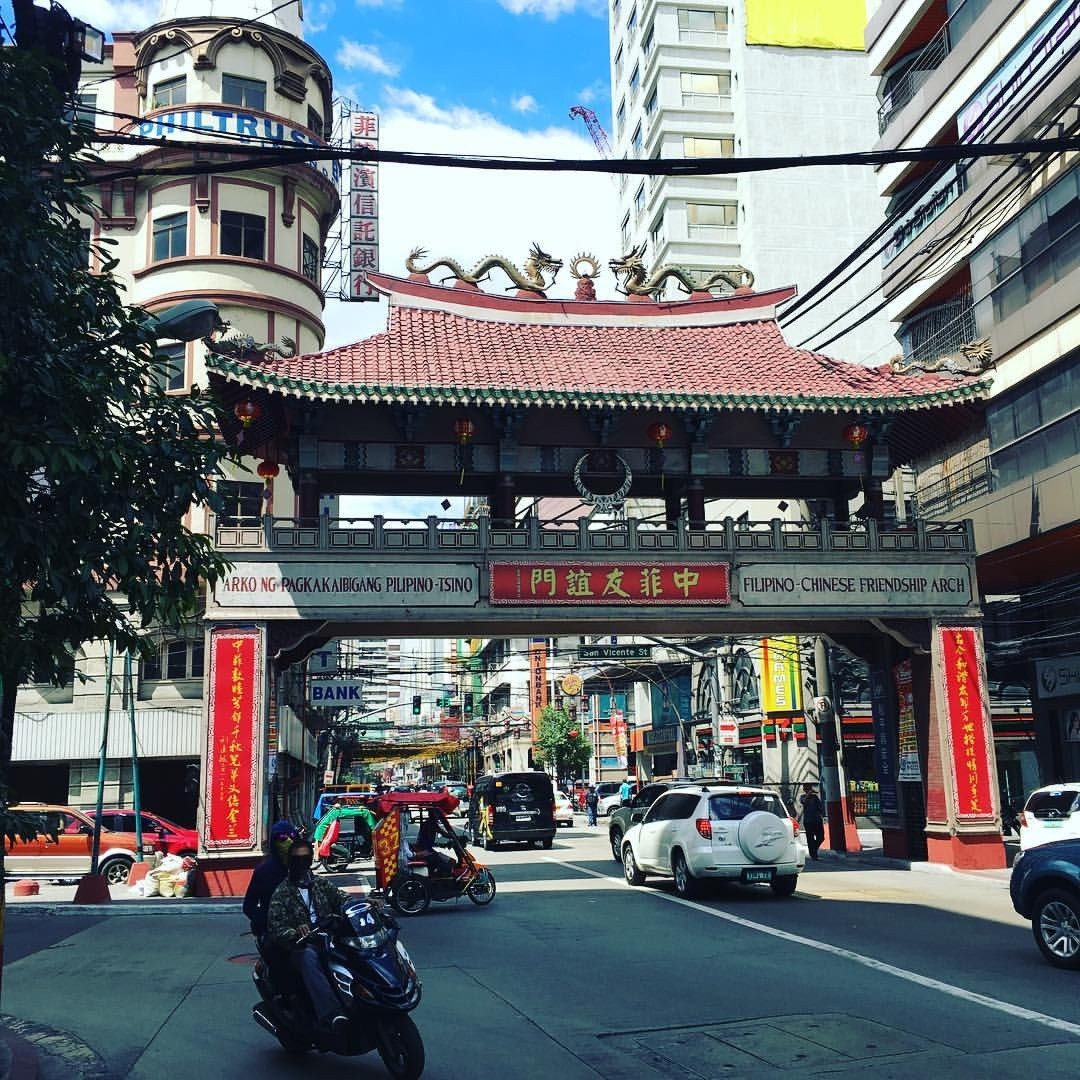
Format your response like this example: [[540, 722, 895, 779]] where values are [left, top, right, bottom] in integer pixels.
[[277, 832, 296, 841]]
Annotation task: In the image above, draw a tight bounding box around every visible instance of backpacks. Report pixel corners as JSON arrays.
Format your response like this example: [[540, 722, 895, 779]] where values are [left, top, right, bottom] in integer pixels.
[[586, 792, 597, 807]]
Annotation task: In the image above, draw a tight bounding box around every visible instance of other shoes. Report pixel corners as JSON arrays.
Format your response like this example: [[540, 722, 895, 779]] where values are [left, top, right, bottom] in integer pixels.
[[330, 1016, 350, 1033]]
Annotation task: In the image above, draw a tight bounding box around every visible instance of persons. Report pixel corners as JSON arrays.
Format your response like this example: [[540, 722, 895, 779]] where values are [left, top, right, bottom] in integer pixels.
[[412, 808, 468, 898], [242, 821, 386, 1047], [616, 779, 632, 809], [798, 783, 825, 861], [584, 784, 600, 827]]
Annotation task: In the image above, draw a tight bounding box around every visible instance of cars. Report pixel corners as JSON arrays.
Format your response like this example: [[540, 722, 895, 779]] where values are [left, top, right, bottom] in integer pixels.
[[1017, 781, 1080, 853], [62, 808, 198, 862], [1008, 837, 1080, 970], [0, 801, 137, 887], [553, 790, 574, 829]]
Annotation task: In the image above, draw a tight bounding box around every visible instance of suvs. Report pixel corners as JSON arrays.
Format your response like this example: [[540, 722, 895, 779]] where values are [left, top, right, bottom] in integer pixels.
[[619, 780, 806, 902], [608, 775, 744, 865]]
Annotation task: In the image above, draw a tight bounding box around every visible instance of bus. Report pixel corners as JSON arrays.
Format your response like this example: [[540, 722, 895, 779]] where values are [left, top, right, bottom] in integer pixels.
[[592, 777, 653, 819]]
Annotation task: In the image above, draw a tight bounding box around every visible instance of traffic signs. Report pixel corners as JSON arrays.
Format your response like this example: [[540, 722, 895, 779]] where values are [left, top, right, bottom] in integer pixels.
[[717, 719, 740, 746]]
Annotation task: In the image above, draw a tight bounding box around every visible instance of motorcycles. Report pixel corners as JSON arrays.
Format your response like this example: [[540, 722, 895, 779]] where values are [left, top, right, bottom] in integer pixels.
[[374, 788, 497, 918], [250, 873, 426, 1080], [311, 779, 470, 873]]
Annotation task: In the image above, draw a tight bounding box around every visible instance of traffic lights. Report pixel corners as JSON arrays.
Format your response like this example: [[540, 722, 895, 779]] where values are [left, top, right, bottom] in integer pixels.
[[444, 699, 448, 708], [413, 695, 421, 715], [464, 693, 474, 720], [806, 655, 819, 697]]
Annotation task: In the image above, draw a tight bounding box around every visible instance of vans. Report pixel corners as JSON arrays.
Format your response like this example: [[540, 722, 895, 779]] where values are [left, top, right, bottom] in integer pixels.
[[464, 769, 559, 852]]
